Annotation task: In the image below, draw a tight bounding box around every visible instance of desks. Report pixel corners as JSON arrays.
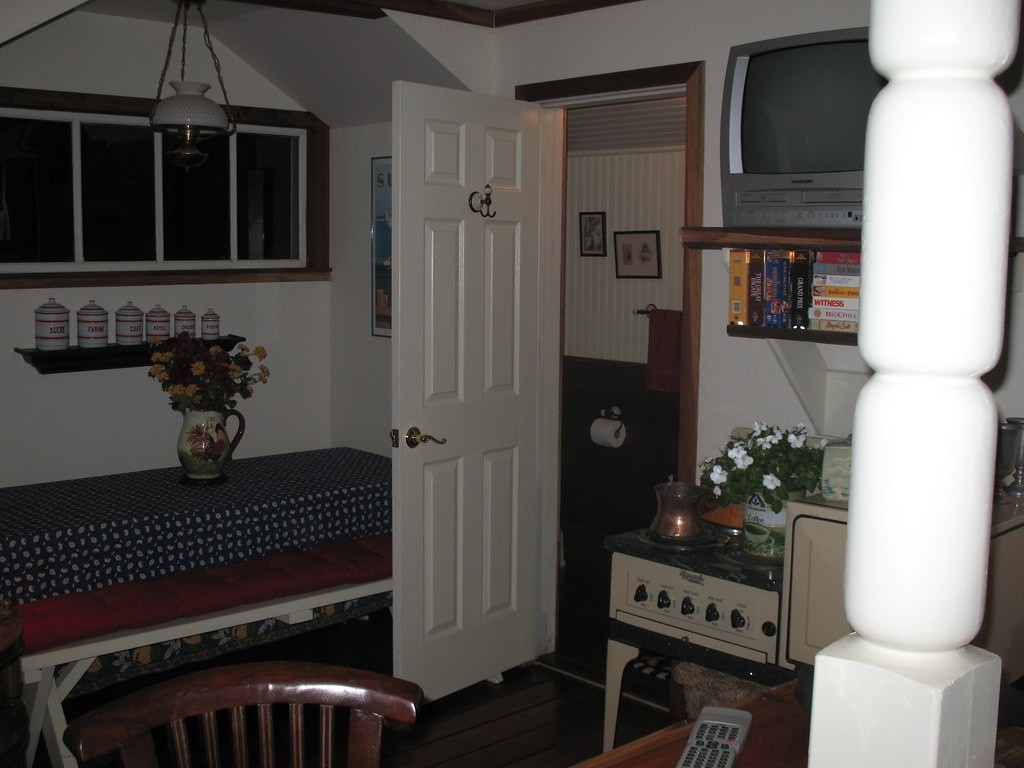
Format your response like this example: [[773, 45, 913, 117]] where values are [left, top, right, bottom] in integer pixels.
[[0, 446, 394, 702], [568, 676, 809, 768]]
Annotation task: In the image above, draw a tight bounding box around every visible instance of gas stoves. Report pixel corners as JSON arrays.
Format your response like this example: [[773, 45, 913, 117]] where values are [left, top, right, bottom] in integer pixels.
[[605, 515, 785, 666]]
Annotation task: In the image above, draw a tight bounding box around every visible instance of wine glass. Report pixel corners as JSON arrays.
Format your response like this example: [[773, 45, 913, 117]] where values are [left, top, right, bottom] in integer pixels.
[[993, 417, 1024, 504]]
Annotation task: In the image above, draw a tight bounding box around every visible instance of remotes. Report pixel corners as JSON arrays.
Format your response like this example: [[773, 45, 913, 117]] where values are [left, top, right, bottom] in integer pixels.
[[674, 706, 753, 768]]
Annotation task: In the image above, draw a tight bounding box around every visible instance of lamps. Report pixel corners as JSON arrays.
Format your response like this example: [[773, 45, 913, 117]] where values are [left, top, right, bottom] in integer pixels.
[[150, 0, 236, 174]]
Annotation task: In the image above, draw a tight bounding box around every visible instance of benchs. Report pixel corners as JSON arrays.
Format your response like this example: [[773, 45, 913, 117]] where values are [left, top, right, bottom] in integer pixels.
[[21, 578, 392, 768]]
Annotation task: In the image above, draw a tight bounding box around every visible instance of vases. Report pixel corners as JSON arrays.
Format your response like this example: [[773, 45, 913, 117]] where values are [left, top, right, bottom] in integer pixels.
[[742, 486, 806, 562], [176, 408, 245, 480]]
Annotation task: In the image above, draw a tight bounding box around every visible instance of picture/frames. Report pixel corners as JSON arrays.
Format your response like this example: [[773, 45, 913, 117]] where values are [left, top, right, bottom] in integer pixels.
[[579, 211, 607, 257], [613, 229, 662, 279], [370, 155, 392, 337]]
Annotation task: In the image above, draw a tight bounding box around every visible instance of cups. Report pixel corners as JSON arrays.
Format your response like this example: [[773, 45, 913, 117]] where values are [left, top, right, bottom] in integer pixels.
[[174, 305, 196, 339], [76, 300, 109, 349], [35, 298, 69, 351], [201, 308, 220, 340], [115, 301, 144, 345], [744, 521, 775, 546], [146, 304, 171, 343]]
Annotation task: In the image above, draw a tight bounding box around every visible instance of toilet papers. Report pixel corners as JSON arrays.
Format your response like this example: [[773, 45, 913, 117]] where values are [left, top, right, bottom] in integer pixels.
[[590, 418, 626, 448]]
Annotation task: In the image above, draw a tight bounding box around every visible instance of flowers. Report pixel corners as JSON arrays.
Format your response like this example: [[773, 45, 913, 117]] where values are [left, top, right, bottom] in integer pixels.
[[146, 330, 270, 411], [698, 418, 827, 511]]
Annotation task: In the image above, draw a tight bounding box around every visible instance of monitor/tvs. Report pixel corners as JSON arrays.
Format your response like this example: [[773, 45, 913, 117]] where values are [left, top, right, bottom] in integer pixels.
[[719, 26, 892, 230]]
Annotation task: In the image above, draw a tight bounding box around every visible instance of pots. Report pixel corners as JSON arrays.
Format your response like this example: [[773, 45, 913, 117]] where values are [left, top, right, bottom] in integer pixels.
[[651, 481, 720, 540]]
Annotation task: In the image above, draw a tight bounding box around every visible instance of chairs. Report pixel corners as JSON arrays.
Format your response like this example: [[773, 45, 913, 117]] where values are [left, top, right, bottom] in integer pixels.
[[62, 663, 425, 768]]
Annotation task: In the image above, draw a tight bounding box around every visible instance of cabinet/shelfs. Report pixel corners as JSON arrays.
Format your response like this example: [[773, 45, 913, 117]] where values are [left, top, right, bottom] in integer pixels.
[[679, 226, 862, 345]]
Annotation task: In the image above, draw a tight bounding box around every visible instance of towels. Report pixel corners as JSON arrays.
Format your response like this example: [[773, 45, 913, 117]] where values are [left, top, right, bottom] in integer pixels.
[[645, 309, 682, 393]]
